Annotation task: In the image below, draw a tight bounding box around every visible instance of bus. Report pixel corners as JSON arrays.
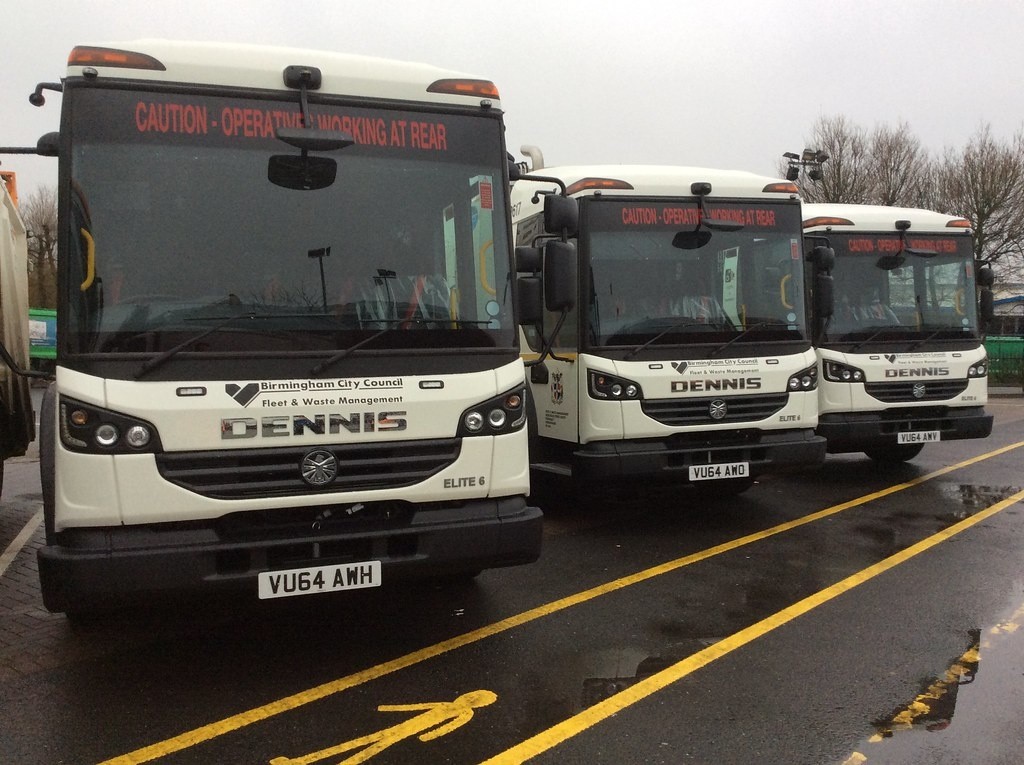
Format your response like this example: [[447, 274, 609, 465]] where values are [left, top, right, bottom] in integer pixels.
[[723, 204, 995, 467], [442, 144, 835, 516], [0, 39, 579, 625]]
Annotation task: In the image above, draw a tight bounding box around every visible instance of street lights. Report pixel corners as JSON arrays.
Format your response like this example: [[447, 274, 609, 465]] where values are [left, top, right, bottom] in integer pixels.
[[307, 247, 332, 315], [782, 148, 829, 198], [374, 267, 397, 306]]
